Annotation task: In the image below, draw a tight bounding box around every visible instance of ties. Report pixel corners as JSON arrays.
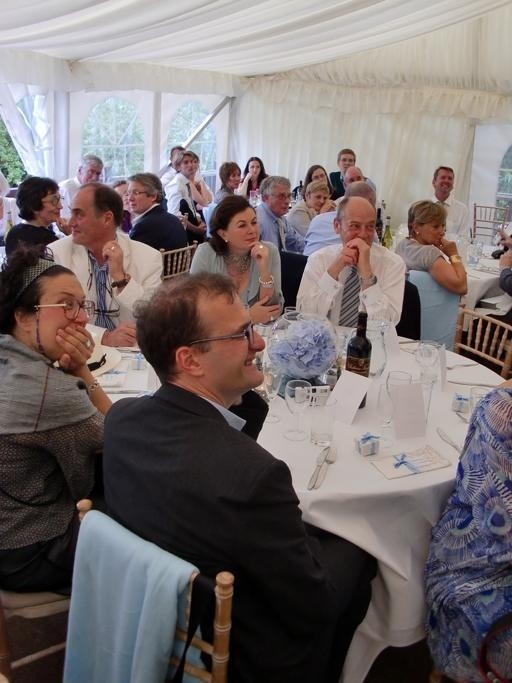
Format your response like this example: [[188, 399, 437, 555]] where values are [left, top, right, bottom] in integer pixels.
[[277, 217, 286, 249], [187, 182, 196, 217], [338, 262, 359, 327]]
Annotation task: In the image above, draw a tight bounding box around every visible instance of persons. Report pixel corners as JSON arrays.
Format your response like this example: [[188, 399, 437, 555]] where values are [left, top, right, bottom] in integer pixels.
[[189, 194, 284, 324], [395, 200, 468, 296], [5, 177, 72, 266], [432, 166, 471, 243], [127, 173, 188, 277], [1, 242, 116, 600], [297, 196, 405, 332], [102, 270, 380, 682], [52, 154, 103, 240], [46, 182, 163, 347], [215, 149, 381, 256], [492, 220, 511, 296], [111, 180, 136, 238], [424, 379, 512, 683], [159, 147, 212, 244]]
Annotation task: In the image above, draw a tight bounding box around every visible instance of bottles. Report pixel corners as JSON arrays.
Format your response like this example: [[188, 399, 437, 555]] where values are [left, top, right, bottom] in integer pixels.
[[374, 207, 384, 238], [381, 216, 392, 247], [345, 310, 373, 407], [297, 179, 303, 199], [251, 350, 273, 398], [5, 210, 14, 238]]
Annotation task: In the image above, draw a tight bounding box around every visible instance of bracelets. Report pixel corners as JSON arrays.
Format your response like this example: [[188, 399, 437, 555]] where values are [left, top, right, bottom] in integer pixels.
[[87, 380, 100, 395], [111, 274, 130, 288], [259, 276, 276, 284]]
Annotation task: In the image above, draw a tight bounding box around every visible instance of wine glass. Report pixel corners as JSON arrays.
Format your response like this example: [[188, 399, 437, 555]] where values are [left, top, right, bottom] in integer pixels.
[[283, 379, 311, 441], [265, 359, 282, 424]]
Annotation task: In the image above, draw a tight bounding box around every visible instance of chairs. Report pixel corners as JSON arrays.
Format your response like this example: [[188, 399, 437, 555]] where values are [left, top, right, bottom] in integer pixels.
[[0, 190, 512, 682]]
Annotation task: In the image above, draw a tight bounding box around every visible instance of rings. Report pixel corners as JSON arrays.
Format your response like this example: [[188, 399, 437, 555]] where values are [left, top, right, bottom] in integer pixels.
[[271, 316, 274, 321], [260, 246, 263, 248], [111, 246, 114, 250]]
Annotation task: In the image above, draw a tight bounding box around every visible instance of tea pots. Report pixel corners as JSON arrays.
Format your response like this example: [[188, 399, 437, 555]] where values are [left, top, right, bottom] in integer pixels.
[[346, 318, 389, 376]]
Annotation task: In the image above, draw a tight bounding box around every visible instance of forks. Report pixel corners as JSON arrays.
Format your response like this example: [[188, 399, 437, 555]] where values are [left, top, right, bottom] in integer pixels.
[[455, 411, 469, 425]]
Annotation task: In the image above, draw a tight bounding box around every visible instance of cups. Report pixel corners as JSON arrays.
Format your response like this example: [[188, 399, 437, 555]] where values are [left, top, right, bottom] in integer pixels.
[[234, 188, 301, 207], [311, 397, 336, 443], [243, 302, 300, 344], [389, 223, 484, 267], [469, 386, 490, 414], [370, 320, 442, 428]]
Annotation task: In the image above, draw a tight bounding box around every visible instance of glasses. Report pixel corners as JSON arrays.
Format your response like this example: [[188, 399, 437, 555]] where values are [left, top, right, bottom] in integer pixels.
[[34, 297, 96, 320], [42, 194, 61, 205], [188, 322, 254, 349], [272, 193, 291, 199], [128, 190, 147, 195]]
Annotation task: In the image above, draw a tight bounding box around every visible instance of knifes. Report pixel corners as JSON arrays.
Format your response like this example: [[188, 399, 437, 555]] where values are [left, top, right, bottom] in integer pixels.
[[434, 426, 461, 452], [308, 445, 329, 488]]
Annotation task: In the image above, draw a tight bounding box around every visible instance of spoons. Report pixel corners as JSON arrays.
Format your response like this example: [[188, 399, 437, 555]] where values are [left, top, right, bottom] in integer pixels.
[[314, 446, 337, 487]]
